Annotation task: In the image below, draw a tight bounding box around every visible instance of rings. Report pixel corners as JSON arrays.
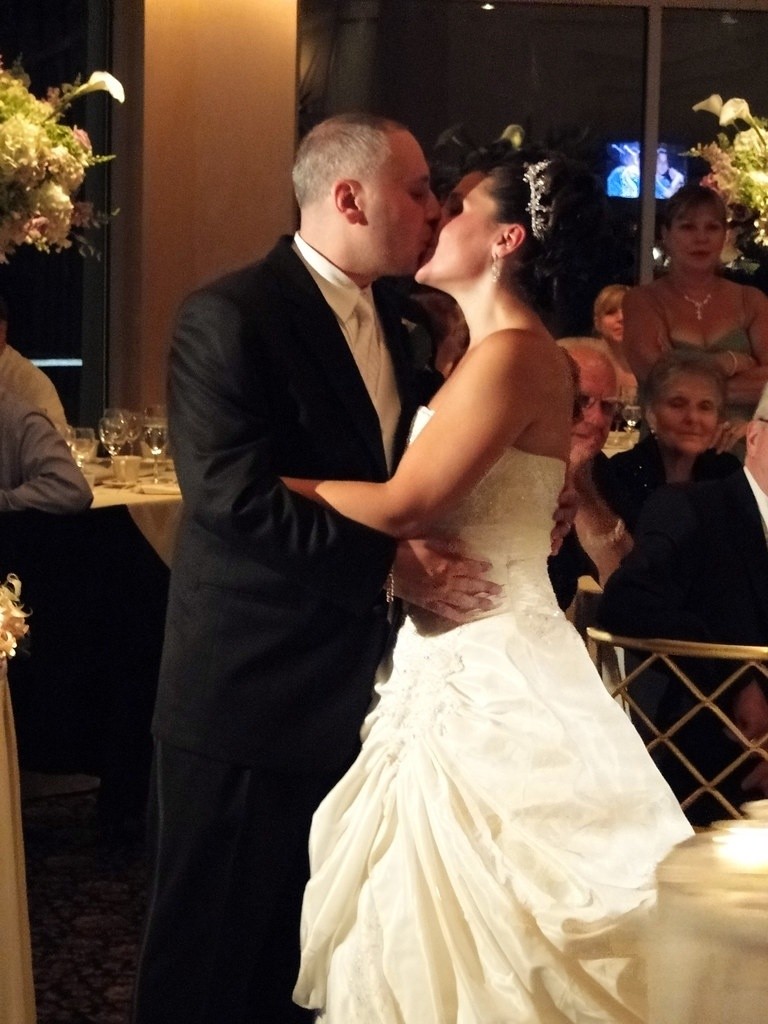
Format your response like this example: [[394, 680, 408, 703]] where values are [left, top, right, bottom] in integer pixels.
[[567, 522, 572, 534]]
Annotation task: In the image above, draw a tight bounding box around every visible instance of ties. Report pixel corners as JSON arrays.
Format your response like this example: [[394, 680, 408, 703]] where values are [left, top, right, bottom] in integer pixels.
[[354, 292, 380, 398]]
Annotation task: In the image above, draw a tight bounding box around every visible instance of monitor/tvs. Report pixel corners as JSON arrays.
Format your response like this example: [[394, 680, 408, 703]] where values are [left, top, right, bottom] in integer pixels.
[[606, 141, 689, 202]]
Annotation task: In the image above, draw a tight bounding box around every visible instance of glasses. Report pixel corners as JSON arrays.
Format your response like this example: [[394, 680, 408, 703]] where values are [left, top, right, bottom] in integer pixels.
[[577, 390, 621, 418]]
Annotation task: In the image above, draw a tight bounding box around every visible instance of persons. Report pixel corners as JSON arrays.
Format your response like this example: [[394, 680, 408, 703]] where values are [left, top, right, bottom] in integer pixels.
[[556, 183, 768, 827], [130, 113, 699, 1024], [607, 143, 684, 198], [0, 308, 99, 514]]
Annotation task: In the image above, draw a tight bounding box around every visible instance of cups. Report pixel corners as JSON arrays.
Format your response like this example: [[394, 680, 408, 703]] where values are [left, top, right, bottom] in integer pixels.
[[103, 408, 129, 420], [112, 456, 140, 483]]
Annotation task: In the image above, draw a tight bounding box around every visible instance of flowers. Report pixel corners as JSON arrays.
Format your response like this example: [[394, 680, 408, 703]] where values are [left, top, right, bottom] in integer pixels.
[[0, 573, 33, 659], [0, 60, 126, 263], [689, 93, 768, 246]]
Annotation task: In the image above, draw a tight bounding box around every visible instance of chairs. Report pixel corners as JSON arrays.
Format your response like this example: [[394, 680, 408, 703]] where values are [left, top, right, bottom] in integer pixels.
[[589, 628, 768, 826], [0, 507, 168, 833]]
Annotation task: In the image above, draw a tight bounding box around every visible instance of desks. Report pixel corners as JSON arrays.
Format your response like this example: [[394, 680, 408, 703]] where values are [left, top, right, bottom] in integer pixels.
[[70, 454, 186, 566]]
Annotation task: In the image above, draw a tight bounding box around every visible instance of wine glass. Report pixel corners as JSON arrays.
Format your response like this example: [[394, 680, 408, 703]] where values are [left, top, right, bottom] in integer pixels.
[[72, 428, 96, 472], [609, 400, 626, 446], [124, 412, 140, 455], [97, 419, 126, 463], [623, 406, 642, 435], [139, 406, 172, 486]]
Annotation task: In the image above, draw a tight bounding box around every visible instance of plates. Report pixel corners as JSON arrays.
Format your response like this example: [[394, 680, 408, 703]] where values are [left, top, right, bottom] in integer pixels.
[[103, 479, 138, 487]]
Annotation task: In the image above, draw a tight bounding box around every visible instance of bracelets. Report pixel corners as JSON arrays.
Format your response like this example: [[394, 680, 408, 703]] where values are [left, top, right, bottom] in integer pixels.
[[385, 564, 395, 603]]
